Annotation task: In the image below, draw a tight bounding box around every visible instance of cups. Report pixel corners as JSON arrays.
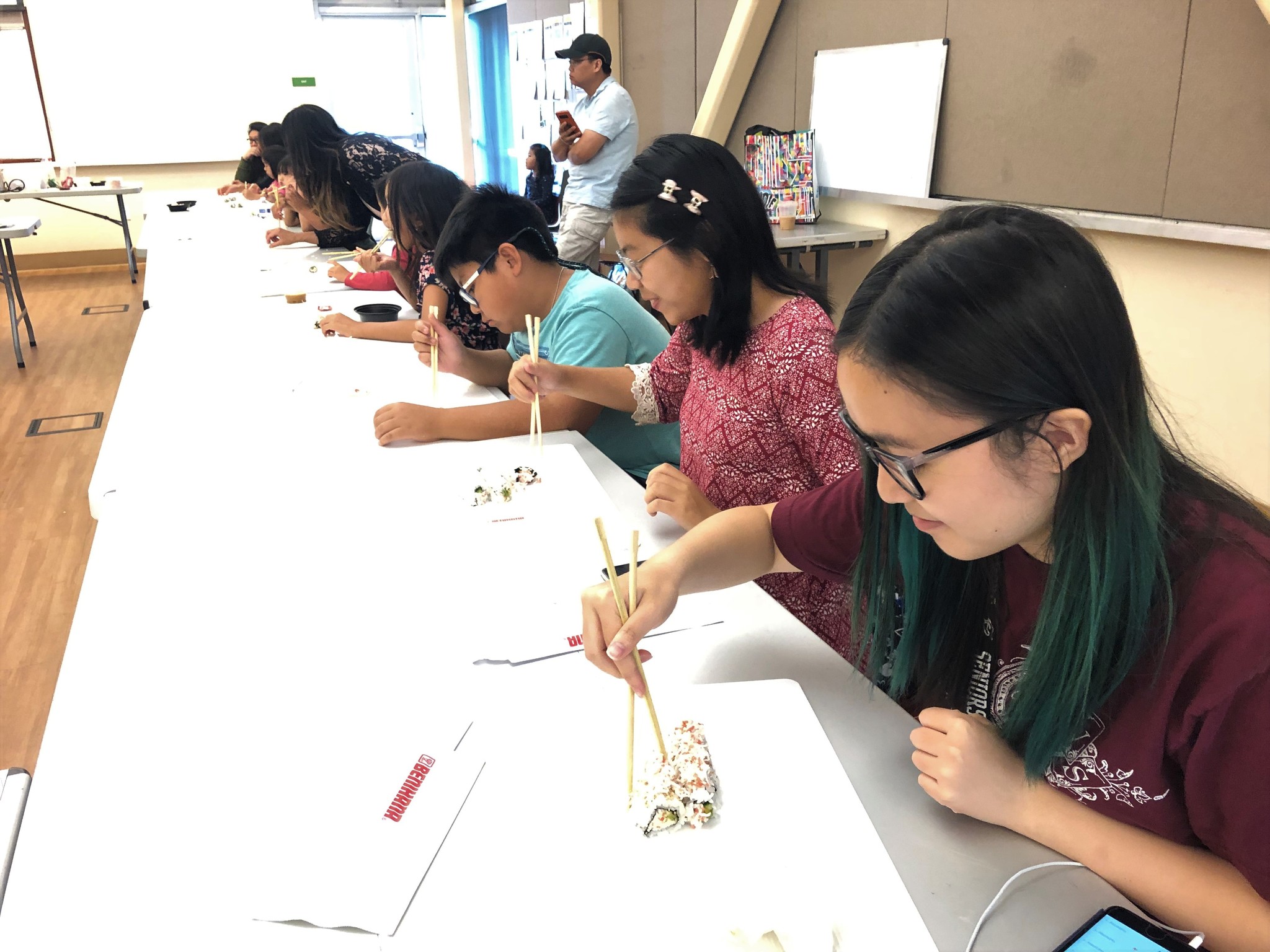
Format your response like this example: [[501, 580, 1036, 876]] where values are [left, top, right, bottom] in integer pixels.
[[284, 288, 306, 304]]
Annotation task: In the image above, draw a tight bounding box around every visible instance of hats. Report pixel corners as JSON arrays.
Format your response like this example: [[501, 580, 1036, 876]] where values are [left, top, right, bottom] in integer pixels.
[[555, 33, 611, 66]]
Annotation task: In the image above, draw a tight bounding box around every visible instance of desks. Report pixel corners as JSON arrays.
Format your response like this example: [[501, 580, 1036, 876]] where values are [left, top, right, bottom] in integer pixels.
[[0, 185, 1153, 952]]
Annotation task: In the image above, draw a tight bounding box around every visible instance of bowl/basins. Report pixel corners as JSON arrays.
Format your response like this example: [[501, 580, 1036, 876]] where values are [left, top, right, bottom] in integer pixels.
[[166, 203, 192, 213], [90, 180, 106, 186], [176, 201, 197, 207], [354, 303, 402, 322]]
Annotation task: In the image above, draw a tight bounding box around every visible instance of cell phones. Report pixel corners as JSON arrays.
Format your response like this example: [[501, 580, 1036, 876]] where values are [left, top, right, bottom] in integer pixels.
[[556, 110, 583, 138], [1052, 906, 1199, 952], [601, 561, 646, 582]]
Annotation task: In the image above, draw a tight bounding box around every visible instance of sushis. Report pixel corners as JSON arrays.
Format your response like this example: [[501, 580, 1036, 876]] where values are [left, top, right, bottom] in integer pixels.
[[472, 463, 539, 506], [635, 721, 721, 838]]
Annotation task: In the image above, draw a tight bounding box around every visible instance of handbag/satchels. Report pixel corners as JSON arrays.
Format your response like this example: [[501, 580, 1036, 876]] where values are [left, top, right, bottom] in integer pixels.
[[743, 124, 822, 226]]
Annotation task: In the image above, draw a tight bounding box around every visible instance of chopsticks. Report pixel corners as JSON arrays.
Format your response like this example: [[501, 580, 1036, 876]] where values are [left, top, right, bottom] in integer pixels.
[[245, 181, 254, 189], [350, 231, 393, 280], [595, 517, 668, 815], [267, 185, 287, 196], [271, 186, 282, 229], [430, 305, 438, 391], [321, 251, 362, 261], [524, 313, 542, 447]]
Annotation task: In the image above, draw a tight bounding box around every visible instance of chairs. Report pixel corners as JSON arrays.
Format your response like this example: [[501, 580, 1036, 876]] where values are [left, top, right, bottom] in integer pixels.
[[547, 170, 569, 227]]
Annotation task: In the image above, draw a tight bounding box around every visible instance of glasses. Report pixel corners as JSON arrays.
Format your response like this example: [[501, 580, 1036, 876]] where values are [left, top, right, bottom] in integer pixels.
[[247, 138, 260, 145], [569, 58, 598, 65], [616, 235, 678, 281], [837, 394, 1064, 501], [459, 232, 519, 308]]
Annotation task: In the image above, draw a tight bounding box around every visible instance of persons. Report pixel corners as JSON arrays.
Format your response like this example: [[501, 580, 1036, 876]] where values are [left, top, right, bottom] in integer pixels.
[[215, 101, 423, 293], [552, 32, 638, 274], [518, 140, 556, 224], [575, 203, 1269, 951], [319, 158, 502, 357], [374, 179, 686, 494], [505, 129, 881, 678]]
